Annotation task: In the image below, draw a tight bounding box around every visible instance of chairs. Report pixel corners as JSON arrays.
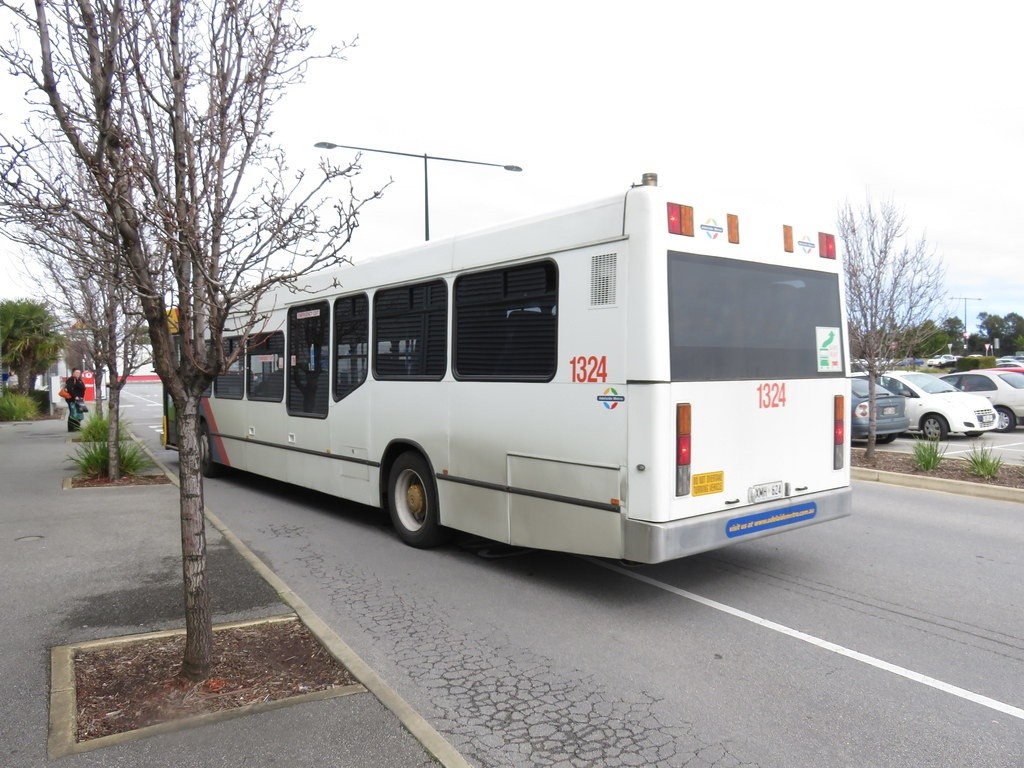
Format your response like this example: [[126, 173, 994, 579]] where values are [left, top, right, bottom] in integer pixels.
[[219, 307, 558, 399]]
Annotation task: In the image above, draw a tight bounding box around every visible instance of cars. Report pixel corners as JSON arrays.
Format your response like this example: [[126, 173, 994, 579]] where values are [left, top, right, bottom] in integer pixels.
[[849, 347, 1024, 446]]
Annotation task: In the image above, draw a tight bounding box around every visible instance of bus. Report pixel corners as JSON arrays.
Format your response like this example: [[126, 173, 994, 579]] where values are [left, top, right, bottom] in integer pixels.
[[160, 165, 857, 566]]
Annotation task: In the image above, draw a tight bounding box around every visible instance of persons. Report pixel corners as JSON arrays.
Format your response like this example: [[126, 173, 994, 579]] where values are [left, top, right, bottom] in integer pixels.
[[62, 367, 89, 433], [6, 371, 19, 388]]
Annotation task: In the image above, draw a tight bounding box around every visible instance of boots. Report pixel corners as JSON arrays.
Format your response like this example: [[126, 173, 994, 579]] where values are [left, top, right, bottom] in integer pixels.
[[68, 418, 81, 432]]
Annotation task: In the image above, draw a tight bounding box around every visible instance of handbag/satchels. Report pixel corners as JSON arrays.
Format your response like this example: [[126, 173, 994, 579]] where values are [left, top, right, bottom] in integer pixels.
[[75, 398, 88, 413], [59, 378, 76, 400]]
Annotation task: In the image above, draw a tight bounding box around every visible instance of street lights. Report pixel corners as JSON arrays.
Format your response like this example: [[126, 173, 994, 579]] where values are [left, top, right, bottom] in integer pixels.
[[314, 139, 525, 241]]
